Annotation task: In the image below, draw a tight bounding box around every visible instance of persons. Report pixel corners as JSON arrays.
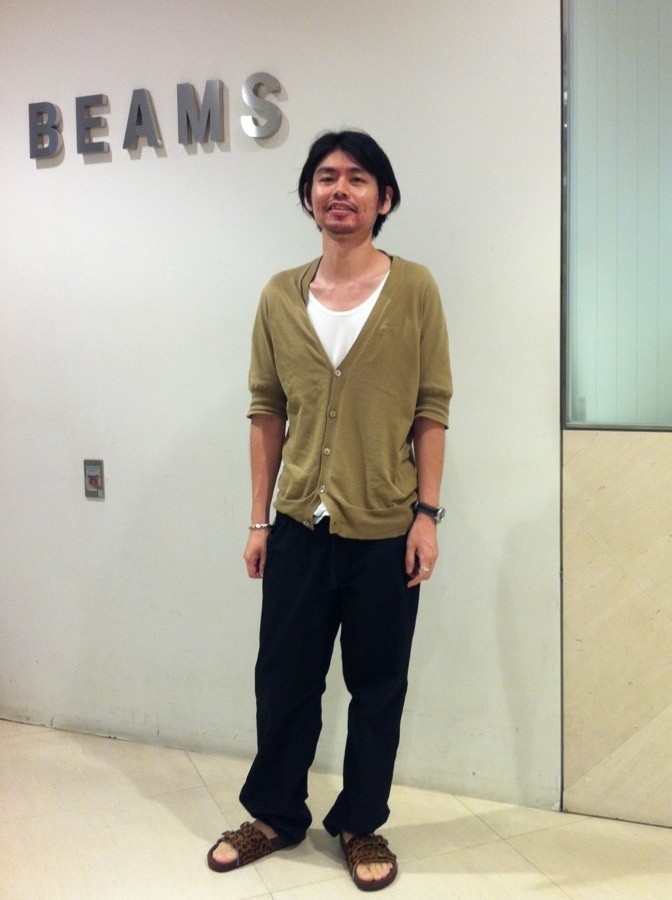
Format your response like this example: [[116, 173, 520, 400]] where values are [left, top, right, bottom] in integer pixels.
[[208, 129, 452, 892]]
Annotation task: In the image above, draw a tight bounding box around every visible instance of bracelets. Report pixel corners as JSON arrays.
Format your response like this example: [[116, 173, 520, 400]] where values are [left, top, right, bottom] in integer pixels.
[[249, 523, 268, 530]]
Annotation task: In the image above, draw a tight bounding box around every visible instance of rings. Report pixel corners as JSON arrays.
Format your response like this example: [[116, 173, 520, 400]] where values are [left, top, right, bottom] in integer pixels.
[[419, 566, 429, 571]]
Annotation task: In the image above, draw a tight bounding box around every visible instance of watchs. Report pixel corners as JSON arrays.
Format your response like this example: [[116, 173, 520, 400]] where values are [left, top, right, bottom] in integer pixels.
[[412, 501, 445, 522]]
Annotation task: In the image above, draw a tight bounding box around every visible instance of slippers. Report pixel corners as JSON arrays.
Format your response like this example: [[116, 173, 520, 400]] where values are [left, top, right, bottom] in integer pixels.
[[207, 821, 291, 873], [340, 832, 397, 891]]
